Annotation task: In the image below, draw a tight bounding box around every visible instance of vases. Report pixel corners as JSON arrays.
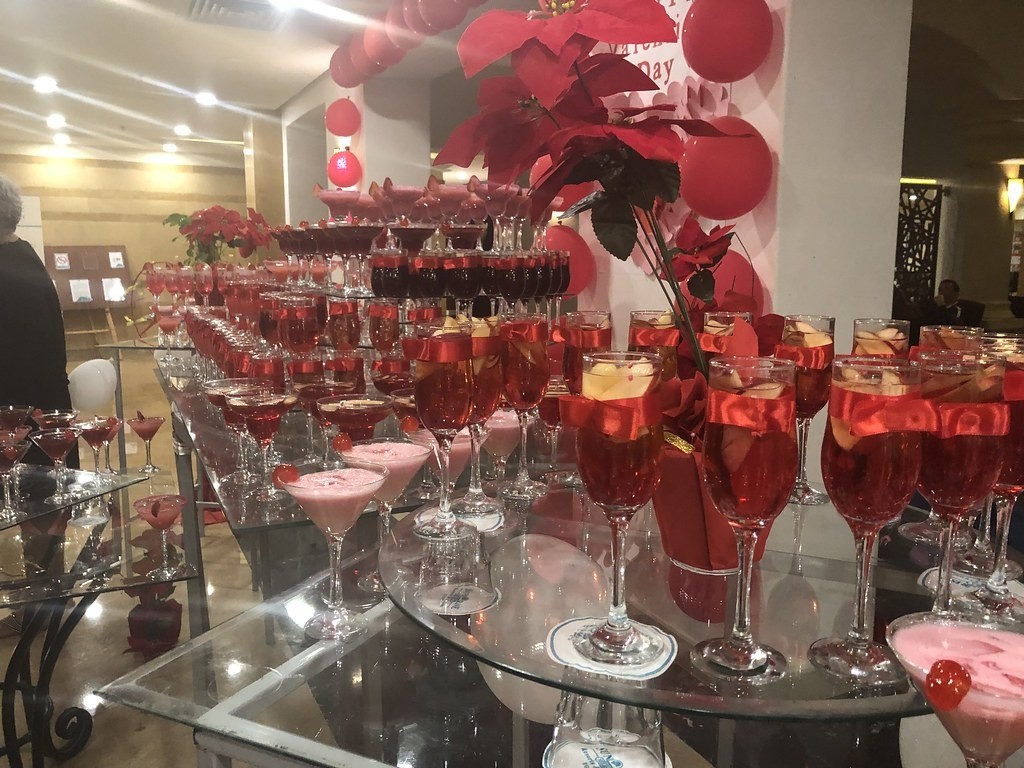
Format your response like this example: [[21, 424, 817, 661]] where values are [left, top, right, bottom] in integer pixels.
[[193, 265, 226, 305]]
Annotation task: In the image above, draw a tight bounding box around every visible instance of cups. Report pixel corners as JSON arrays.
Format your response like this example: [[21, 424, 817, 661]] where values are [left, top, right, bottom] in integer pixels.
[[418, 530, 497, 615], [548, 664, 667, 768]]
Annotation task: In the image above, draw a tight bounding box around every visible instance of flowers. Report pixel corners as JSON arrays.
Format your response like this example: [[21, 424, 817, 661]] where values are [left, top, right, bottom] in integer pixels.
[[164, 204, 270, 273]]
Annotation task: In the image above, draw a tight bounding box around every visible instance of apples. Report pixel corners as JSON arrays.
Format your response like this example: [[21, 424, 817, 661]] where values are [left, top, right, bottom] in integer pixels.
[[283, 310, 1024, 469]]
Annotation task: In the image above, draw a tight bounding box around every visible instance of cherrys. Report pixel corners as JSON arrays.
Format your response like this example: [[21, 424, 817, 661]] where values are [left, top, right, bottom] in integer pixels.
[[924, 660, 972, 712]]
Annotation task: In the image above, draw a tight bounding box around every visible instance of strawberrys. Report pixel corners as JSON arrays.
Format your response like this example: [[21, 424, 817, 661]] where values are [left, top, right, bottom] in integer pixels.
[[151, 500, 160, 517], [4, 409, 145, 459]]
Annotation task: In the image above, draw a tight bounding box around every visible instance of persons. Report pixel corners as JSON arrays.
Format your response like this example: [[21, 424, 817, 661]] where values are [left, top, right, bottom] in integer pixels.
[[934, 279, 972, 326], [0, 177, 81, 640]]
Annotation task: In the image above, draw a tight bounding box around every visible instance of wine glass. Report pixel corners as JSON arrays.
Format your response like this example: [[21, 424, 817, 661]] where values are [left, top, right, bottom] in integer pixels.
[[69, 416, 124, 491], [127, 417, 165, 475], [0, 404, 83, 524], [277, 461, 391, 640], [885, 611, 1024, 768], [571, 309, 1024, 685], [337, 437, 434, 591], [133, 494, 187, 582], [146, 178, 611, 519]]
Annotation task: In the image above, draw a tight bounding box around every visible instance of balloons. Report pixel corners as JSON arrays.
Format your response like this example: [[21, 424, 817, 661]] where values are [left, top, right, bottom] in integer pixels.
[[328, 147, 362, 187], [677, 116, 773, 220], [326, 98, 361, 137], [682, 0, 772, 83], [67, 357, 120, 411], [532, 220, 593, 301], [330, 0, 490, 88], [529, 155, 592, 211], [674, 249, 765, 337]]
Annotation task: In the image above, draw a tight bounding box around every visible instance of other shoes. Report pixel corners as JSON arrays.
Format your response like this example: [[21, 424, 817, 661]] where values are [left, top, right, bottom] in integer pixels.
[[4, 588, 75, 608], [0, 610, 52, 638]]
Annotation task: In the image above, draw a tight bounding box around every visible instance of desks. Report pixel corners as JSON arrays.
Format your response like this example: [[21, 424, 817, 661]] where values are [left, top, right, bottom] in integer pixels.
[[0, 313, 1024, 768]]
